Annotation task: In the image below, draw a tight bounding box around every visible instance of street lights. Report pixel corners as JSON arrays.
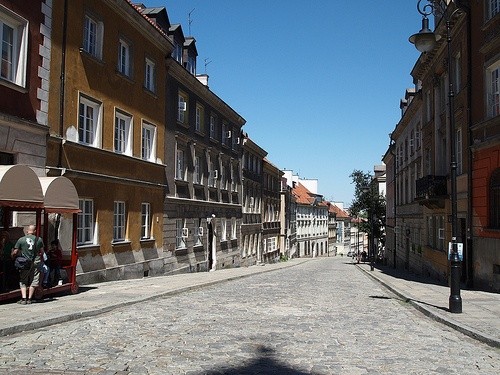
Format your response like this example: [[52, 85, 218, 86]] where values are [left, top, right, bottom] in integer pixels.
[[408, 0, 465, 313]]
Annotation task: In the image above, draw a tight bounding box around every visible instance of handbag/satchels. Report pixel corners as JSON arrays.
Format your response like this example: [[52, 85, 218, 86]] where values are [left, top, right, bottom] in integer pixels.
[[14, 257, 30, 271], [59, 268, 68, 283]]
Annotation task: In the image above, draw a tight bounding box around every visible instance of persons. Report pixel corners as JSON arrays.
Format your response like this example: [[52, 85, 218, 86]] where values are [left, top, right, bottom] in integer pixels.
[[0, 224, 50, 305], [48, 239, 63, 286], [360, 251, 367, 264]]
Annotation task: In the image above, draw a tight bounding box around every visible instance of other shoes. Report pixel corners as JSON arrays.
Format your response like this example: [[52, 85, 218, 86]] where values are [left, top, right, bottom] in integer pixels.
[[17, 300, 27, 304], [57, 280, 63, 287], [47, 282, 54, 288], [26, 300, 31, 304], [42, 284, 48, 289]]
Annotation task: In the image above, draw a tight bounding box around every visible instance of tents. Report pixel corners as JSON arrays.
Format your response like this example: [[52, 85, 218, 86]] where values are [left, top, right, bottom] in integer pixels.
[[0, 164, 82, 301]]
